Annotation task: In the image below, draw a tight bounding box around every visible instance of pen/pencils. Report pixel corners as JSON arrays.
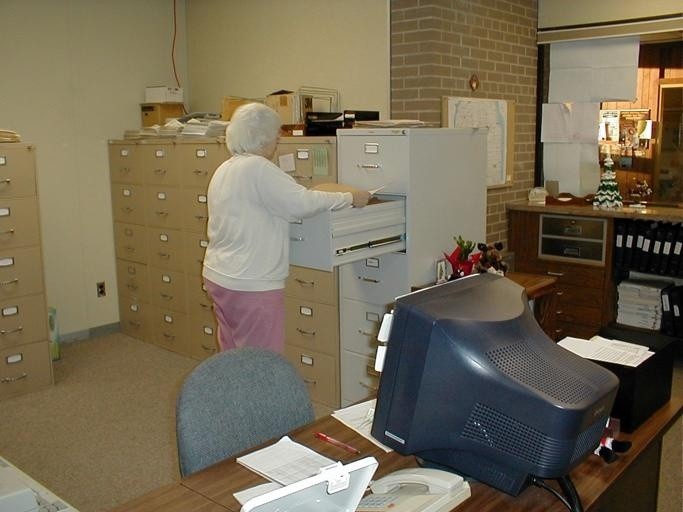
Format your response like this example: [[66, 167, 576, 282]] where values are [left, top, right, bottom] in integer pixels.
[[444, 251, 456, 265], [314, 431, 361, 456]]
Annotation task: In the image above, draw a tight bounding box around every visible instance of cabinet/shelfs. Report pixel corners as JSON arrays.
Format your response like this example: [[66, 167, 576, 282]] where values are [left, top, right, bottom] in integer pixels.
[[108, 126, 489, 409], [0, 141, 54, 401], [505, 209, 616, 344]]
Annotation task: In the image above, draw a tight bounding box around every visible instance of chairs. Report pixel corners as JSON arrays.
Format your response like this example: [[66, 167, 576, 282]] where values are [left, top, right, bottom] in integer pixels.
[[174, 344, 320, 488]]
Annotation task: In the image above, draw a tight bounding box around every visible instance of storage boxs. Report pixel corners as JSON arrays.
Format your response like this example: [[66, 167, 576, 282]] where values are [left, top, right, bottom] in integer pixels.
[[139, 84, 183, 127]]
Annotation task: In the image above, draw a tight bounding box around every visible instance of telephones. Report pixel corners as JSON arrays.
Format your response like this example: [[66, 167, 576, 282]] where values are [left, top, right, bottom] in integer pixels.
[[355, 467, 471, 512]]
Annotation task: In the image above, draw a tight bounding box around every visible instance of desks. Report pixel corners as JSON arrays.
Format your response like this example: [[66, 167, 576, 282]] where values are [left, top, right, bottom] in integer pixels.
[[108, 395, 683, 512]]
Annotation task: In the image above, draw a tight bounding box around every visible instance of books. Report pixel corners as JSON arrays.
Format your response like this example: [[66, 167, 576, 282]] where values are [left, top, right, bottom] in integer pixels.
[[616, 280, 674, 330]]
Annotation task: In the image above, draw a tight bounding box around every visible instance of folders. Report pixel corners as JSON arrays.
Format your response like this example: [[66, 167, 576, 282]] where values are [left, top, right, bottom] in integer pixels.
[[661, 285, 683, 339], [614, 218, 683, 278]]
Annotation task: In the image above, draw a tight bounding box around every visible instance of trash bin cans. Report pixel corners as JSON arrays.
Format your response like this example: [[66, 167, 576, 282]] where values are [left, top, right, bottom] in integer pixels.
[[47, 306, 59, 360]]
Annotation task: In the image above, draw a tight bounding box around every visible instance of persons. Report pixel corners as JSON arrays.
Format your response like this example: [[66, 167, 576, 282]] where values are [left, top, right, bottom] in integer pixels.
[[202, 101, 374, 355]]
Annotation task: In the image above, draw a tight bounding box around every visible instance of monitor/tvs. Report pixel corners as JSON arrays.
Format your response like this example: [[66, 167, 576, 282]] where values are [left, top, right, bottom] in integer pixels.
[[371, 273, 619, 497]]
